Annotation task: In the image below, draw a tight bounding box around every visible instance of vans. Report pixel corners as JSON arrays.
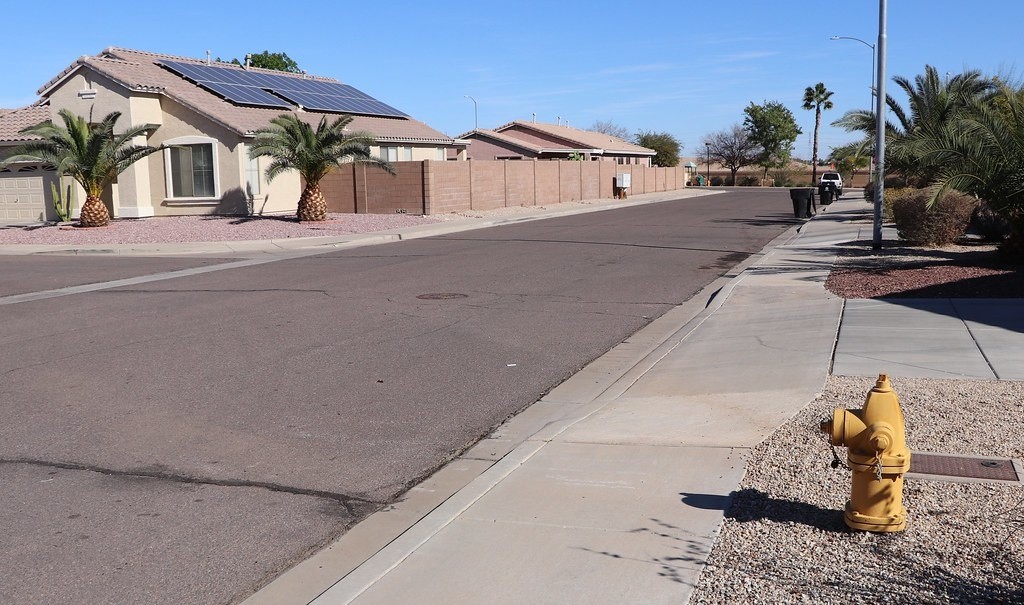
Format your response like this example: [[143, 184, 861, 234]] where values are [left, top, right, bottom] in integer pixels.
[[820, 173, 843, 194]]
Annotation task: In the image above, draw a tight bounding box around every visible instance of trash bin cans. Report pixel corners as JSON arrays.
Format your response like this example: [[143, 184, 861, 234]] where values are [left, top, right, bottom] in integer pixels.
[[790, 188, 817, 218], [818, 184, 839, 205]]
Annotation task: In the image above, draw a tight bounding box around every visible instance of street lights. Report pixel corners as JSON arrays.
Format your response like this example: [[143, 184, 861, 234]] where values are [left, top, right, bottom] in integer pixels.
[[464, 94, 478, 129], [705, 142, 711, 181], [829, 34, 876, 184]]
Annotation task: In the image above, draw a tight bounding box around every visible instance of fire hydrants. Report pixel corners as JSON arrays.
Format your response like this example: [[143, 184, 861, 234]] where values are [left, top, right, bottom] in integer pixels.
[[818, 373, 913, 533]]
